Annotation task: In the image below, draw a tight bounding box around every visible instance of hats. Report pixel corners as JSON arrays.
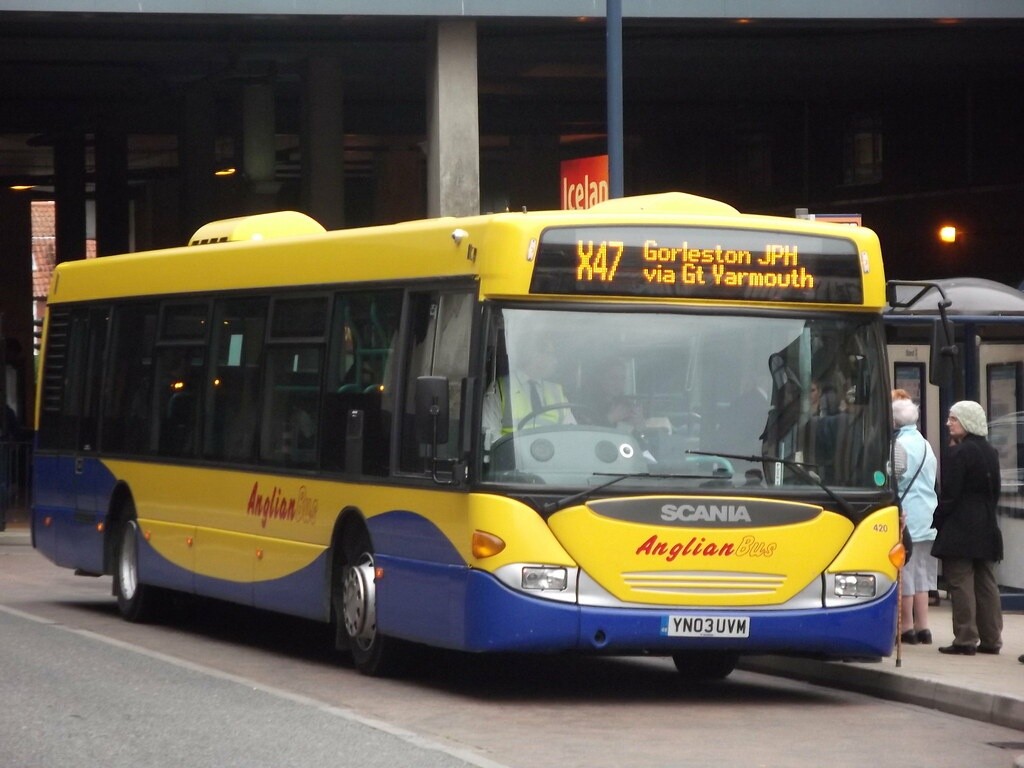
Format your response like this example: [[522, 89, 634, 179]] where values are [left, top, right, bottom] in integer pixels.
[[949, 400, 988, 436]]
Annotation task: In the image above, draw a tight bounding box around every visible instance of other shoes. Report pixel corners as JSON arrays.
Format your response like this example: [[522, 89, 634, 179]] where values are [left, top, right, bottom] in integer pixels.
[[1018, 654, 1024, 663]]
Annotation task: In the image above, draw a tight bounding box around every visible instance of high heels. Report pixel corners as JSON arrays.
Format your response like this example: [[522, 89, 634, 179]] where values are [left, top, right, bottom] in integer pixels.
[[896, 629, 916, 644], [916, 628, 932, 644]]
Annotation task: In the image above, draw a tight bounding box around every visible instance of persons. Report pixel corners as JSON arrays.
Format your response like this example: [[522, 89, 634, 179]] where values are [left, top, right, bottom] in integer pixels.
[[588, 371, 648, 446], [930, 401, 1005, 655], [810, 372, 857, 482], [891, 399, 938, 645], [891, 389, 910, 402], [481, 331, 578, 463], [0, 388, 26, 530], [344, 358, 378, 384]]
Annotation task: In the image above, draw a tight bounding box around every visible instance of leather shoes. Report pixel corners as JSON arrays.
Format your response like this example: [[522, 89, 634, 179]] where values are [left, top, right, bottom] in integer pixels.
[[976, 644, 1000, 655], [938, 644, 977, 656]]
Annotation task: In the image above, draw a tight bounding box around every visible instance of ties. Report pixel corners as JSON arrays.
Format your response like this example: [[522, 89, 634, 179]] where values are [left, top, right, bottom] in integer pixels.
[[526, 380, 543, 412]]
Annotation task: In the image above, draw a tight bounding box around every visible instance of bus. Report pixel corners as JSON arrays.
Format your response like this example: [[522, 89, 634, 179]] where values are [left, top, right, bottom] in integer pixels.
[[26, 189, 963, 681]]
